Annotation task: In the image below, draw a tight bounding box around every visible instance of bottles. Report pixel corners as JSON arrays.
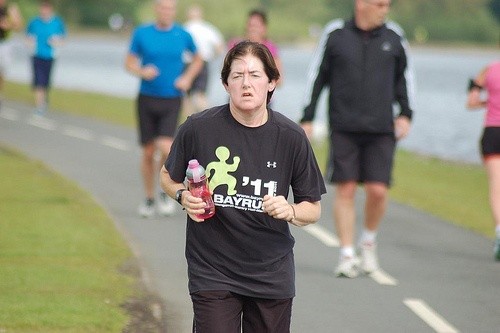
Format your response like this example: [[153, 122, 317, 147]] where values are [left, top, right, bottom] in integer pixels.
[[185, 159, 216, 219]]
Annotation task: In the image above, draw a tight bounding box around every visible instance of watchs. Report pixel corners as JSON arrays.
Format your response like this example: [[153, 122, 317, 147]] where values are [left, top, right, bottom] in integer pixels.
[[175, 189, 188, 212]]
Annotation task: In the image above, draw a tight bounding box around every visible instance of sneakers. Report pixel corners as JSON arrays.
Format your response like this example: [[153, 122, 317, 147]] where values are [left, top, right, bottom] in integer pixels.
[[157, 192, 175, 215], [358, 255, 378, 273], [335, 255, 361, 279], [492, 229, 500, 261], [139, 198, 154, 217]]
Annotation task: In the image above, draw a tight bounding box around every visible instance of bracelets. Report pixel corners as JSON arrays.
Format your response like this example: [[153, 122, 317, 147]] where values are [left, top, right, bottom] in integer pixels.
[[287, 203, 297, 223]]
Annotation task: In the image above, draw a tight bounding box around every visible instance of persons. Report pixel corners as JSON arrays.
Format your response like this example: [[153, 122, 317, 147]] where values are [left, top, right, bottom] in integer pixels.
[[0, 0, 24, 68], [181, 6, 226, 117], [125, 0, 208, 221], [466, 56, 500, 262], [226, 12, 285, 87], [160, 40, 328, 333], [25, 2, 69, 119], [298, 0, 417, 281]]
